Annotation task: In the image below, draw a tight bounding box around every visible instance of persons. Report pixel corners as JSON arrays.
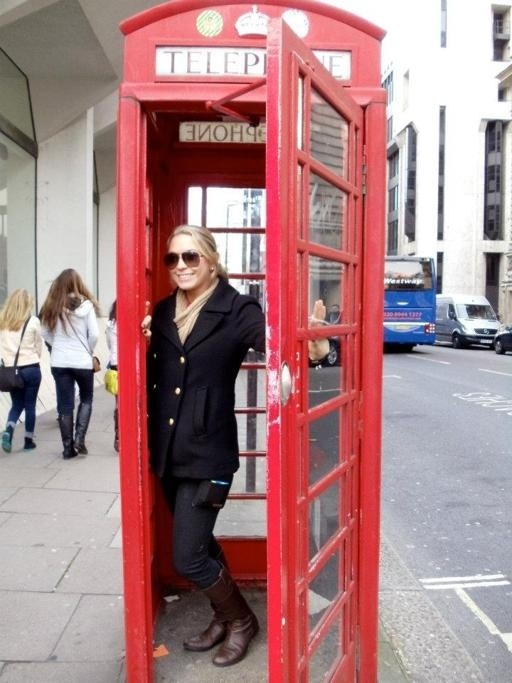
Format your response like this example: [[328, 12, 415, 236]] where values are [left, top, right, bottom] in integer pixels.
[[103, 298, 120, 451], [0, 287, 42, 453], [37, 267, 100, 459], [327, 304, 341, 323], [141, 224, 329, 666]]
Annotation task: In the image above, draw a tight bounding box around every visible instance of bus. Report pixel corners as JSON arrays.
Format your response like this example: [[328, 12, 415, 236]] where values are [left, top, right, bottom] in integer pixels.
[[385, 255, 436, 353]]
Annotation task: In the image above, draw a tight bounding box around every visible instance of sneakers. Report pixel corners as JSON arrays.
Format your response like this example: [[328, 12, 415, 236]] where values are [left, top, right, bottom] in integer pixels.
[[2, 428, 11, 452], [24, 437, 36, 449]]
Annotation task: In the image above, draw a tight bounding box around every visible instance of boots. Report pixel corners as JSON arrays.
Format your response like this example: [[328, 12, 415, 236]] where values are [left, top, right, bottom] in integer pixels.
[[183, 551, 259, 666], [73, 403, 91, 454], [59, 416, 77, 457]]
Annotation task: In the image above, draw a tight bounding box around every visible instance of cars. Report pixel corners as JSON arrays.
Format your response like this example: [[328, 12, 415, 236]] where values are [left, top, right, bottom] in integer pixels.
[[310, 319, 341, 368], [493, 324, 511, 354]]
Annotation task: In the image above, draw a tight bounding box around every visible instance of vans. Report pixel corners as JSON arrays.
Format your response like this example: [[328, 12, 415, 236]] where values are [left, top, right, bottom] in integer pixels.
[[436, 293, 505, 349]]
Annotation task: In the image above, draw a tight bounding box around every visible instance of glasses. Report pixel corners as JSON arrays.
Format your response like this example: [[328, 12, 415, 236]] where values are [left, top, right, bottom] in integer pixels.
[[164, 252, 203, 269]]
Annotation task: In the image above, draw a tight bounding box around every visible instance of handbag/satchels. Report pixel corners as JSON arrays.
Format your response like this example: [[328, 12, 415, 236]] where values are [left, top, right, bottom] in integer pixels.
[[105, 369, 118, 395], [0, 367, 24, 391], [93, 357, 101, 372]]
